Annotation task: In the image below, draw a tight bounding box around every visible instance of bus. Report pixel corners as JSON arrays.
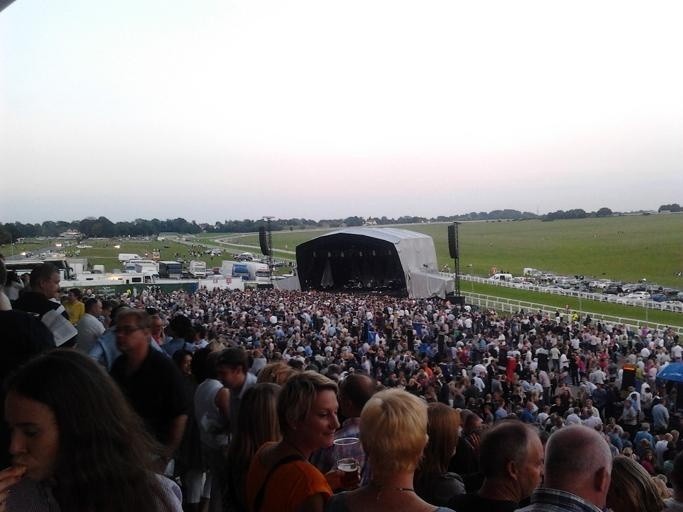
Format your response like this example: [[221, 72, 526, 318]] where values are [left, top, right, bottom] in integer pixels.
[[3, 258, 75, 283]]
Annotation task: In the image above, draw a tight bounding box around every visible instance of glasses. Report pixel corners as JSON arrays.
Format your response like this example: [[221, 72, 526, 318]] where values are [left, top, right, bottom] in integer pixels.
[[113, 325, 145, 336]]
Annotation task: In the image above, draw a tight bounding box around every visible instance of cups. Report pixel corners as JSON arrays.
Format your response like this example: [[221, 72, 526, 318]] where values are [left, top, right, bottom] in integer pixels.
[[333, 437, 361, 491]]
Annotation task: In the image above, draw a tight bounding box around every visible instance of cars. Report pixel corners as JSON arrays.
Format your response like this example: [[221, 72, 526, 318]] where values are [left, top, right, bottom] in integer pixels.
[[483, 264, 682, 312], [21, 240, 81, 260]]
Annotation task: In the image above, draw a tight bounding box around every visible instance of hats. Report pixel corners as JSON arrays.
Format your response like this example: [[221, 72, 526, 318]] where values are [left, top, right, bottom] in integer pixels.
[[296, 346, 348, 353]]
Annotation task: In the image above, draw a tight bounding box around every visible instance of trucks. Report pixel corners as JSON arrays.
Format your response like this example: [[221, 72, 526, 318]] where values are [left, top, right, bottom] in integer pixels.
[[82, 249, 285, 282]]
